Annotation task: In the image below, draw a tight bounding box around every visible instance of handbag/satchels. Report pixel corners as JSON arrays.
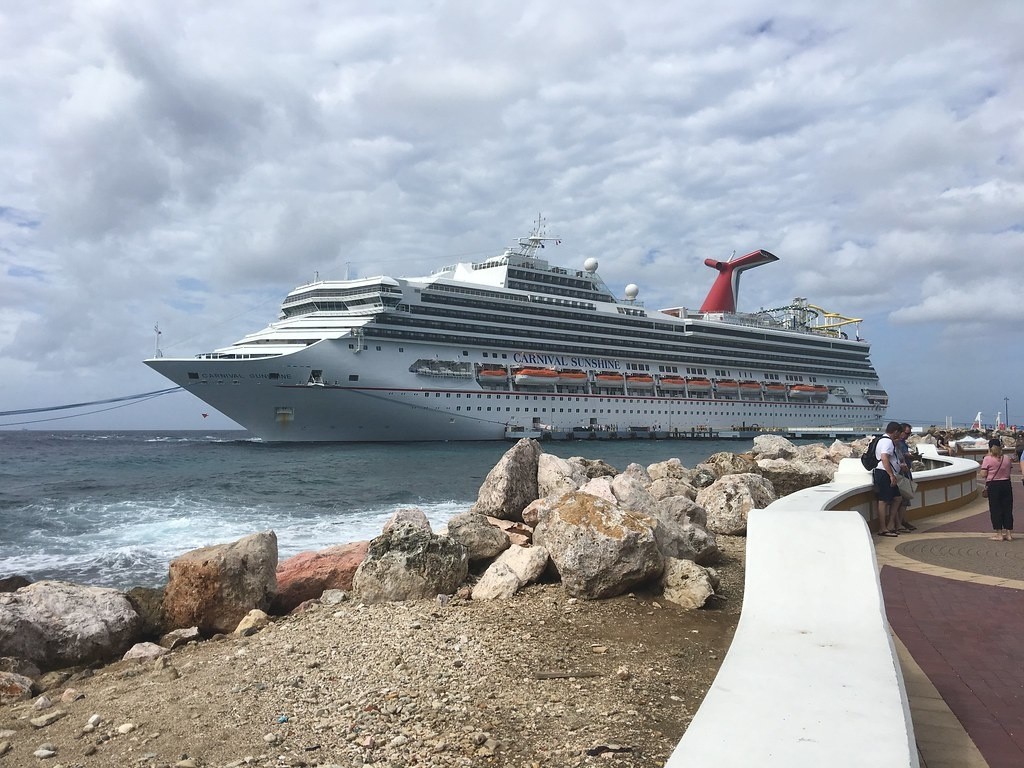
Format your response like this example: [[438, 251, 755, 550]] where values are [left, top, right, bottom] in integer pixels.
[[895, 472, 918, 500], [982, 486, 988, 497]]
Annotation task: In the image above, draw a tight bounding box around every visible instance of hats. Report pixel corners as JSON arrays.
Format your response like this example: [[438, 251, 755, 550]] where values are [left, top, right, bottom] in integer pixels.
[[988, 439, 1000, 448]]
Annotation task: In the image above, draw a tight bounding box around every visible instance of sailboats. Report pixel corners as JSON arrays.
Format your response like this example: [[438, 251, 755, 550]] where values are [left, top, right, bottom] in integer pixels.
[[969, 411, 987, 434], [991, 412, 1002, 434]]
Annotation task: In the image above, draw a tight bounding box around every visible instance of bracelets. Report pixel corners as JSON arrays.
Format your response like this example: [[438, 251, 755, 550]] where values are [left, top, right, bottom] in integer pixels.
[[889, 474, 895, 477]]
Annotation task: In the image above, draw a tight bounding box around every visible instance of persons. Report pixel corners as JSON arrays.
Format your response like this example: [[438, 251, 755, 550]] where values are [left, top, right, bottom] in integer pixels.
[[981, 439, 1015, 541], [871, 421, 903, 537], [937, 431, 952, 456], [982, 431, 1024, 486], [895, 423, 923, 533]]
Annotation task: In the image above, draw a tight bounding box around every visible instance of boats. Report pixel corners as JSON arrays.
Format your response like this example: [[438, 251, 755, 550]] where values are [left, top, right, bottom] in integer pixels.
[[988, 423, 994, 431]]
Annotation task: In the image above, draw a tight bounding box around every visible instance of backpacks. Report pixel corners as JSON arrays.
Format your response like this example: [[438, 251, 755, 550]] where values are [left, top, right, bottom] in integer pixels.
[[861, 436, 890, 471]]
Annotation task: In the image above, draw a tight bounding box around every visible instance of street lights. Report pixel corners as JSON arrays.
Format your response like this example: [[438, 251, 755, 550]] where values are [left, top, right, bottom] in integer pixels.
[[1004, 396, 1010, 427]]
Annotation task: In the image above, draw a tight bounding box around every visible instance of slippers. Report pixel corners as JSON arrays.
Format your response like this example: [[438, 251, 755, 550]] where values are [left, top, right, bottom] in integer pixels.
[[878, 530, 900, 536], [897, 523, 917, 533]]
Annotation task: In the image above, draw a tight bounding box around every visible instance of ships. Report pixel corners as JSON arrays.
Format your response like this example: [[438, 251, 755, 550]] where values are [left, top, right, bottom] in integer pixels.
[[139, 211, 891, 441]]
